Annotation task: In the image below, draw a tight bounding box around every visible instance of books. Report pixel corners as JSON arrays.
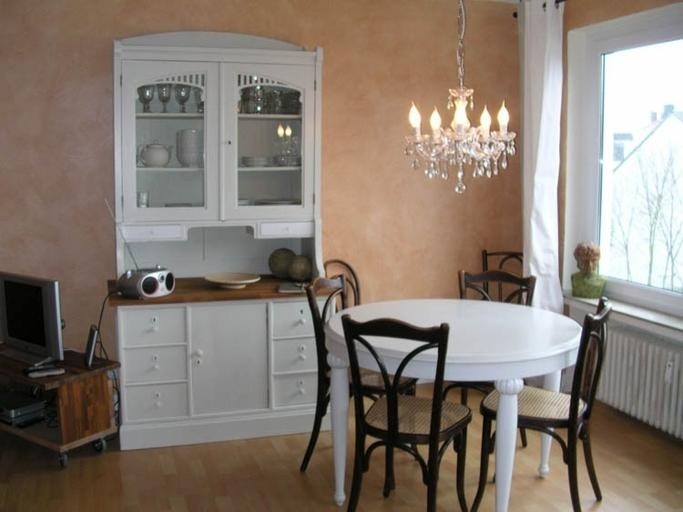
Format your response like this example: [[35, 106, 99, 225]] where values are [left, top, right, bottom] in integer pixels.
[[278, 281, 307, 294]]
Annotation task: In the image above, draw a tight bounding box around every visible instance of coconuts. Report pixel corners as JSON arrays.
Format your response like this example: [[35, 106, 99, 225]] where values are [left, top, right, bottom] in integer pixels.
[[269, 248, 296, 277], [288, 255, 312, 281]]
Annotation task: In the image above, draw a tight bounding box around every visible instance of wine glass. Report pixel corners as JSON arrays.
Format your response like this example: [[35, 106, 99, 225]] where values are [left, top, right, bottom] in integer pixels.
[[140, 84, 204, 113]]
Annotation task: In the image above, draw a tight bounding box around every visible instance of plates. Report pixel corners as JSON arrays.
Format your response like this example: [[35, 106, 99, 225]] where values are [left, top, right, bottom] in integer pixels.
[[241, 154, 299, 166], [255, 199, 300, 204]]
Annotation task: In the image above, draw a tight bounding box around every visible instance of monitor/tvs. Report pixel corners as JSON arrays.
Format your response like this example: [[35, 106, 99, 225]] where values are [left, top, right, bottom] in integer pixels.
[[0, 271, 65, 366]]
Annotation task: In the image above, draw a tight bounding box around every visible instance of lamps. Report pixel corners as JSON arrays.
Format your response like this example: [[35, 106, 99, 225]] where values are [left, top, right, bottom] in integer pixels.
[[402, 0, 517, 195]]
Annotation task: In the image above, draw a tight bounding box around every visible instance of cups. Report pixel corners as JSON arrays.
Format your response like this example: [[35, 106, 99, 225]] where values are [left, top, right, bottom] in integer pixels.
[[136, 191, 150, 208]]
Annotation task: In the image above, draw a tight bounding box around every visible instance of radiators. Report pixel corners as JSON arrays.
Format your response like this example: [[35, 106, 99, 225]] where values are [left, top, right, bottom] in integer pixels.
[[595, 315, 682, 441]]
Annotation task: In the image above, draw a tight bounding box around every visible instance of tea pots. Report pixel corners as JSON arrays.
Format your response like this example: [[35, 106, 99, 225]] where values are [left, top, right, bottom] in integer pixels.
[[136, 139, 175, 166]]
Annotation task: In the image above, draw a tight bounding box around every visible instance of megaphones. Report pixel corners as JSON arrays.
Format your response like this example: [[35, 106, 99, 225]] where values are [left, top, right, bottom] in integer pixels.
[[119, 265, 175, 300]]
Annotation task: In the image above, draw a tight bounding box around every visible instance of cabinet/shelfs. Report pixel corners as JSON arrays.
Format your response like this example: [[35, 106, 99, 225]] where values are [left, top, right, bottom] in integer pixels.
[[105, 27, 348, 451]]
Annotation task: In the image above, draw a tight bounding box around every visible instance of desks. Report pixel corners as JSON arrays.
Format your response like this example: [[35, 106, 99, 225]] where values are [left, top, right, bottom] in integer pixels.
[[0, 345, 119, 465], [318, 295, 586, 507]]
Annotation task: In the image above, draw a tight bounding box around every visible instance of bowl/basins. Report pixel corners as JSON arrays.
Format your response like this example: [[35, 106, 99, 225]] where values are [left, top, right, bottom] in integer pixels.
[[267, 89, 300, 114], [178, 129, 203, 166]]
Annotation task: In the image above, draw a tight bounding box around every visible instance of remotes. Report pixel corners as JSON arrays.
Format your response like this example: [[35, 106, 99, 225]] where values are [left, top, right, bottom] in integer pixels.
[[29, 368, 65, 378]]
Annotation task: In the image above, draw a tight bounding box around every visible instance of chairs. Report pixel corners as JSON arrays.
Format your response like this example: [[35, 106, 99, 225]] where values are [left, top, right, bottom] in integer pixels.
[[333, 313, 475, 512], [478, 248, 528, 309], [470, 294, 607, 512], [446, 267, 538, 485], [320, 255, 366, 312], [297, 272, 424, 498]]
